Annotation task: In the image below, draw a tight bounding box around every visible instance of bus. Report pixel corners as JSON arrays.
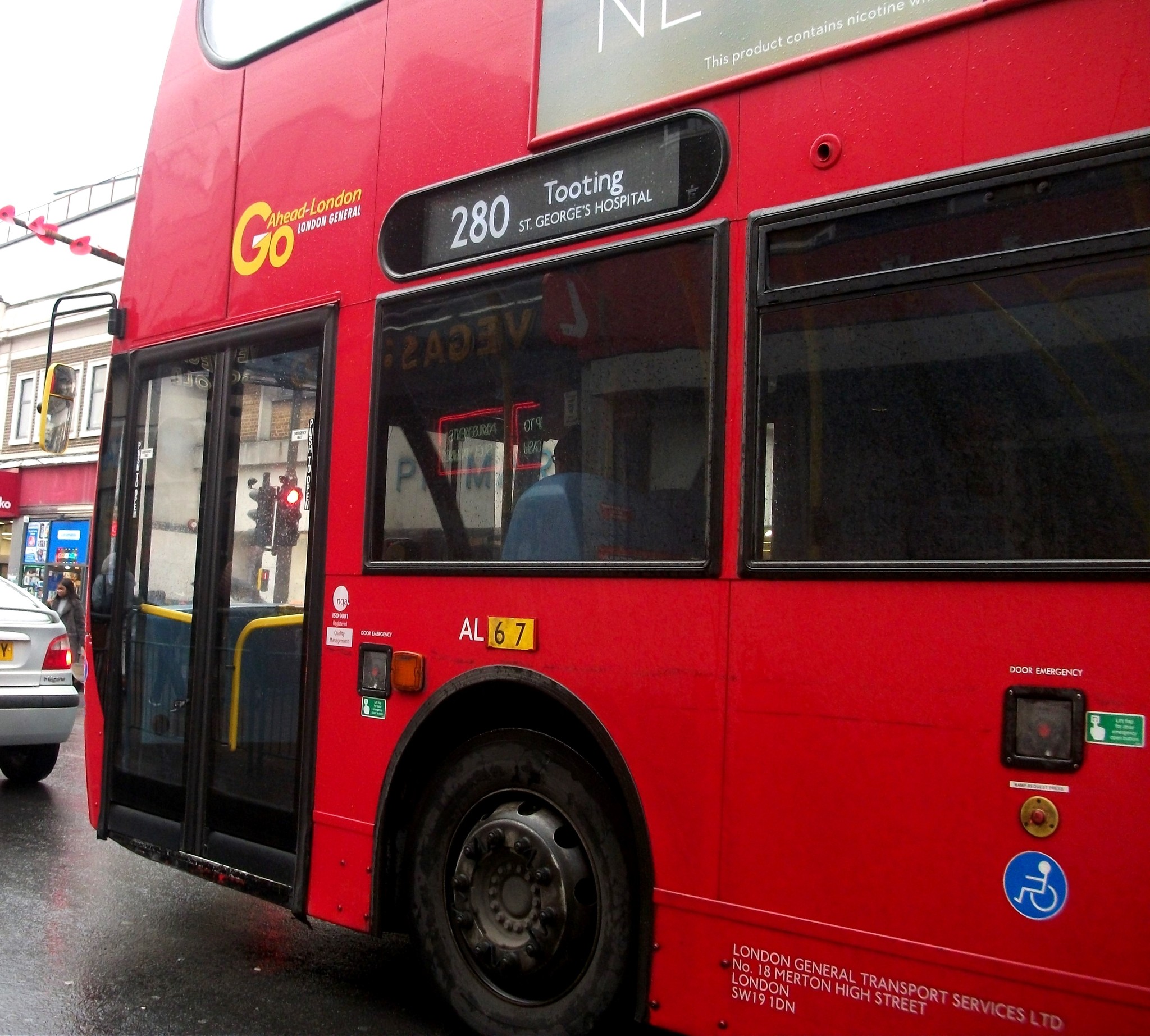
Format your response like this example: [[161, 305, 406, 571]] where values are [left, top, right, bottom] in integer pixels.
[[31, 0, 1150, 1036]]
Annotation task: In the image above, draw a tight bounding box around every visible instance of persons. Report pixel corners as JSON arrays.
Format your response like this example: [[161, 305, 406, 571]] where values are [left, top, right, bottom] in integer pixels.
[[91, 552, 134, 692], [551, 423, 581, 475], [44, 579, 86, 691]]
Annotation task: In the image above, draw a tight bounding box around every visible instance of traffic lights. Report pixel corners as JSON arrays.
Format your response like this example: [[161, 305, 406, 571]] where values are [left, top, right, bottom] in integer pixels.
[[246, 486, 277, 548], [273, 484, 304, 549]]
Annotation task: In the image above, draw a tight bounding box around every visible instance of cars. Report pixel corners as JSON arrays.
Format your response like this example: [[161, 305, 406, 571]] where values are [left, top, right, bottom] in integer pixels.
[[0, 572, 80, 788]]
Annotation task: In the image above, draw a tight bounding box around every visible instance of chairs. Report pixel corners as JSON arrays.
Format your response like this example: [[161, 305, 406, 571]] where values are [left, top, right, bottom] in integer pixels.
[[501, 471, 698, 561]]
